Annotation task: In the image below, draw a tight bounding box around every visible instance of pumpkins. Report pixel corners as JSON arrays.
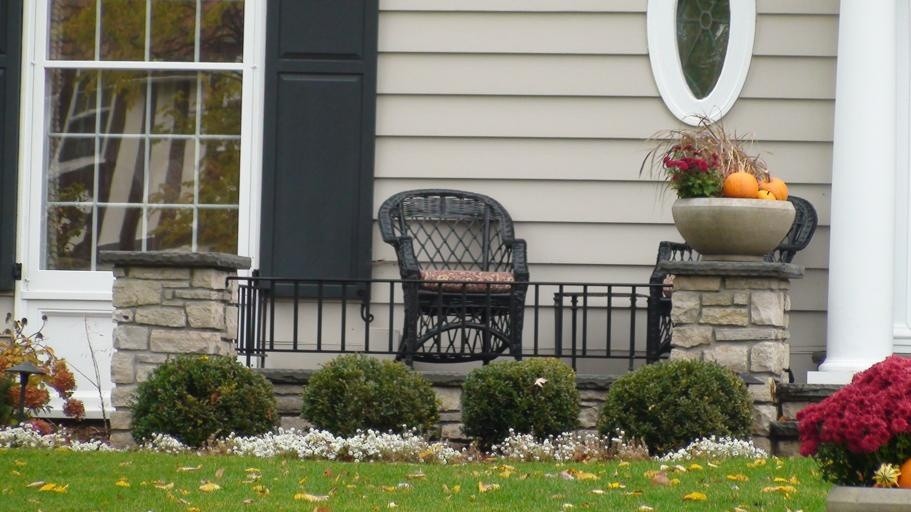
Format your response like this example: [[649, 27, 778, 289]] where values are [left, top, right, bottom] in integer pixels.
[[758, 190, 777, 200], [897, 459, 911, 489], [759, 175, 788, 200], [724, 172, 758, 197]]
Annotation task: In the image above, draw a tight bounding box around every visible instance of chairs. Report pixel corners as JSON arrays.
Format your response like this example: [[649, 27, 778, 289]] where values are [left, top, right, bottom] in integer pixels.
[[375, 188, 529, 367], [647, 193, 817, 363]]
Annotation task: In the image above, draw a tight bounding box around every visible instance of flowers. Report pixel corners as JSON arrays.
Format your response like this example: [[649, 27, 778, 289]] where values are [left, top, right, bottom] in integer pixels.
[[638, 104, 772, 198], [797, 353, 911, 488]]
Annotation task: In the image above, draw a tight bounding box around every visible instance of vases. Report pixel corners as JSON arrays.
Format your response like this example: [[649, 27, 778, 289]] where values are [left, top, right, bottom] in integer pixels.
[[826, 486, 911, 512], [671, 198, 798, 263]]
